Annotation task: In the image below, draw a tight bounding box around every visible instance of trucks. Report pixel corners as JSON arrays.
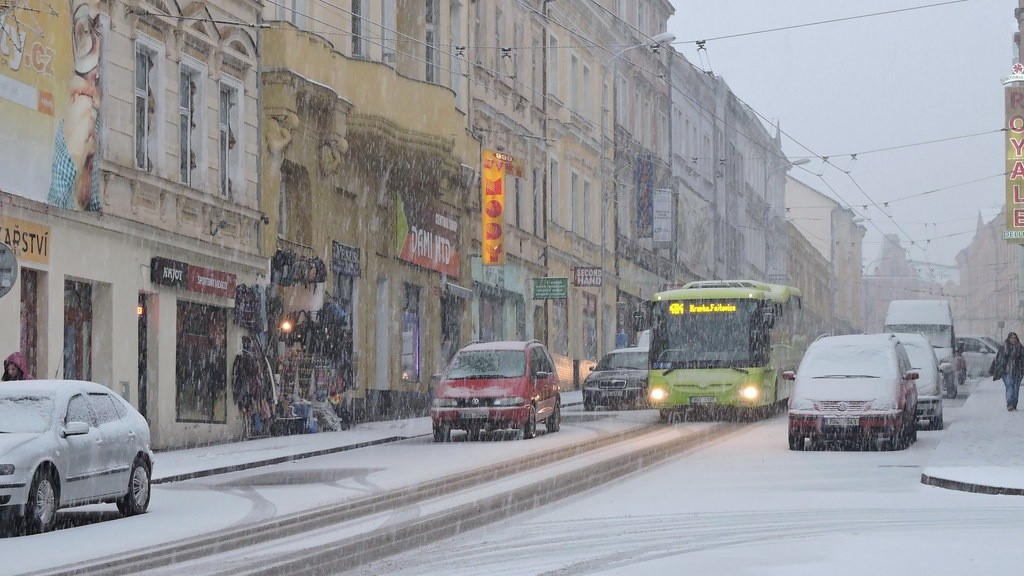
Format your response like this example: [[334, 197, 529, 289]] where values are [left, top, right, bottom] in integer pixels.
[[883, 298, 958, 399]]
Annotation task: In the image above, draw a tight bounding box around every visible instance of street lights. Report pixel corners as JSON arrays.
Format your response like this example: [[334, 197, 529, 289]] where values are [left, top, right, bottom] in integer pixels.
[[831, 217, 865, 334], [599, 32, 676, 362], [763, 158, 811, 282], [866, 256, 890, 333]]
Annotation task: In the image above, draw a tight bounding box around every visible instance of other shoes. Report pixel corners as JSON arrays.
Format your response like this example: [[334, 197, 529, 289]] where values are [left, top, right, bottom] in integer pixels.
[[1007, 405, 1013, 411], [1012, 404, 1016, 409]]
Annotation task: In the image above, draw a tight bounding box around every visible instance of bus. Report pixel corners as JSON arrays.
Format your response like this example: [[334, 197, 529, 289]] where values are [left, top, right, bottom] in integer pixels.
[[647, 279, 803, 422]]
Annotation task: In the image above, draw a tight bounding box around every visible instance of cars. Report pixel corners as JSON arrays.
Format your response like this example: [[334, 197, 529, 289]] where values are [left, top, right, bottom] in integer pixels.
[[955, 340, 966, 385], [955, 334, 999, 381], [891, 333, 943, 430], [0, 380, 155, 535], [583, 348, 649, 411], [977, 335, 1001, 350]]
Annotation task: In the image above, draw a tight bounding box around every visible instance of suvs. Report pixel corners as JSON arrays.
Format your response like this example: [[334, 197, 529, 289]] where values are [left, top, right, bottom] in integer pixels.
[[431, 338, 561, 441], [782, 332, 920, 451]]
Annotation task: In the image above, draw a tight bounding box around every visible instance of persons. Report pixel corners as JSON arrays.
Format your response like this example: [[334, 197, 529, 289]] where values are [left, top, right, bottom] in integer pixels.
[[988, 332, 1024, 411], [46, 0, 103, 216], [1, 352, 36, 381]]
